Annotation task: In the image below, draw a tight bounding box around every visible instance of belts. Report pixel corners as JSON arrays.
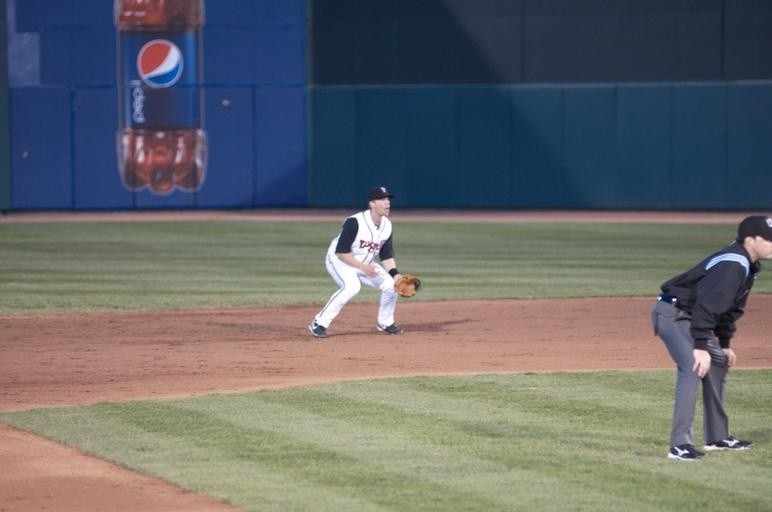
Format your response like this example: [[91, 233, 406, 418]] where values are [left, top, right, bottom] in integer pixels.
[[658, 294, 683, 311]]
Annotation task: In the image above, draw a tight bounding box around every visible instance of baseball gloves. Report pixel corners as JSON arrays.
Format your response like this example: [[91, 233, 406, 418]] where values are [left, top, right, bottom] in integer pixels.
[[395, 275, 420, 296]]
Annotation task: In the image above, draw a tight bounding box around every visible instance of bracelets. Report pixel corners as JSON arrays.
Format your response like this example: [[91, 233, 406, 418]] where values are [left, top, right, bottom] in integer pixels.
[[388, 268, 399, 278]]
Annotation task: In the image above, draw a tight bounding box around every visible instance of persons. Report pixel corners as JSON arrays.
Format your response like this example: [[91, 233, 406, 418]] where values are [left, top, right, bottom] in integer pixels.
[[649, 211, 772, 462], [307, 185, 423, 338]]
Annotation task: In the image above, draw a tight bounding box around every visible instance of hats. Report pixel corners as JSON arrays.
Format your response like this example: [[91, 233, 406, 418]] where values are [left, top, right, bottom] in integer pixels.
[[737, 216, 772, 242], [368, 187, 394, 201]]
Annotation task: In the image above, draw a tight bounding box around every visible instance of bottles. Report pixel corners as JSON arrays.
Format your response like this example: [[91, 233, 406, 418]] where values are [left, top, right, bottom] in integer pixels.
[[114, 0, 208, 194]]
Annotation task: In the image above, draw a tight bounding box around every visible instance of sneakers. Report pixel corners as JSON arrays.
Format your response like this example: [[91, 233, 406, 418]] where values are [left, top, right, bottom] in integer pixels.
[[308, 321, 327, 338], [667, 445, 708, 461], [704, 435, 755, 450], [376, 324, 403, 335]]
